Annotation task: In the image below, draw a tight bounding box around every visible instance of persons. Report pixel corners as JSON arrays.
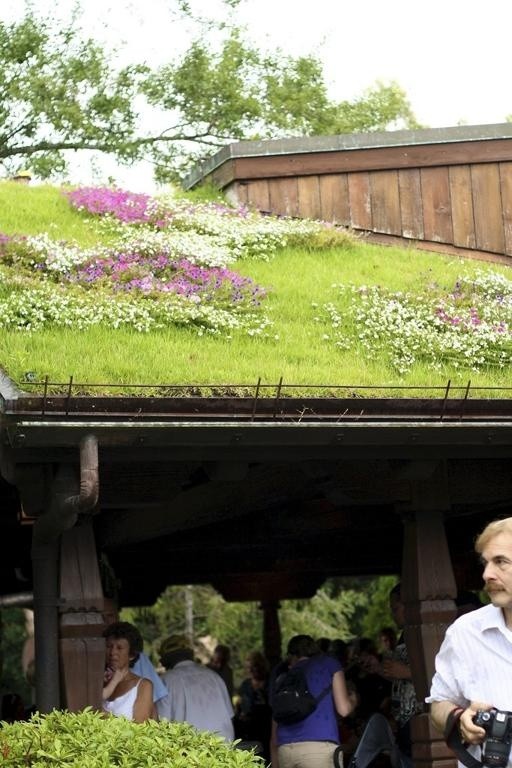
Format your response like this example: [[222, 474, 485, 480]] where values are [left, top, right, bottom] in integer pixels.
[[425, 515, 512, 768], [103, 585, 415, 768]]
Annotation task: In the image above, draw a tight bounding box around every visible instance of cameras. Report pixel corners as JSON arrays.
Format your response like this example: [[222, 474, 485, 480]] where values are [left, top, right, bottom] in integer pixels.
[[475, 707, 512, 768]]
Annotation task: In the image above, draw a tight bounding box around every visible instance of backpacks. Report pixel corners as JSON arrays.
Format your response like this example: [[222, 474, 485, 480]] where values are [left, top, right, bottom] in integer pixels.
[[272, 661, 333, 728]]
